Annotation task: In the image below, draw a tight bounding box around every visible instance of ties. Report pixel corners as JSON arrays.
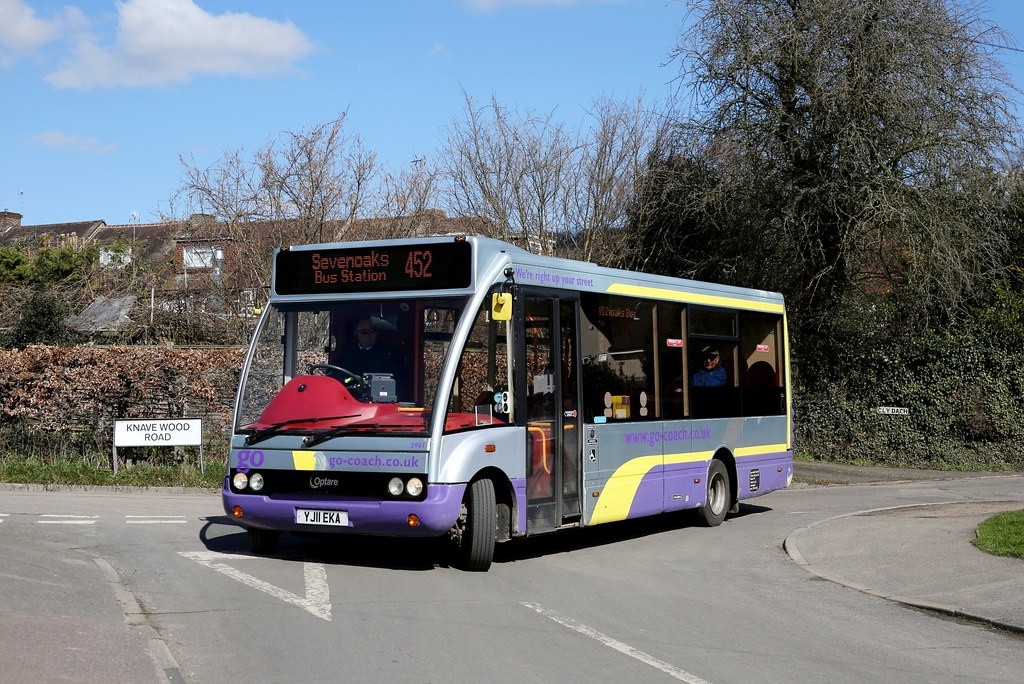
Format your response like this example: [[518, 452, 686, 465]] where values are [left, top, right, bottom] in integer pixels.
[[361, 347, 368, 352]]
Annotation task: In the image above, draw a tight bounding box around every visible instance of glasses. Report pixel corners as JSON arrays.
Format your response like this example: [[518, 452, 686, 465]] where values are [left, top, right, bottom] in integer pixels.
[[356, 328, 376, 335]]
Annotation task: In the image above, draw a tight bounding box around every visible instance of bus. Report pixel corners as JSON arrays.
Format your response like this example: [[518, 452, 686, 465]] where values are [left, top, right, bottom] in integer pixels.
[[222, 234, 796, 572]]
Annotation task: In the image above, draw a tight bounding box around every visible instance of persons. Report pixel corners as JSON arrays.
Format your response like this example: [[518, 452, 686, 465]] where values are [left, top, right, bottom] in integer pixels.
[[691, 345, 728, 387], [332, 317, 395, 402]]
